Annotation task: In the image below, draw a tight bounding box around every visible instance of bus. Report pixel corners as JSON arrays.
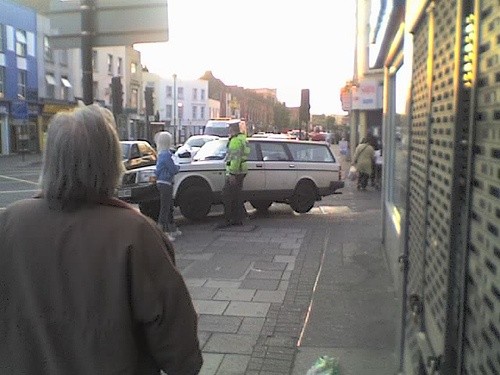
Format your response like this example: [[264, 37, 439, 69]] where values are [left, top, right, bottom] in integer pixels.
[[206, 119, 241, 138]]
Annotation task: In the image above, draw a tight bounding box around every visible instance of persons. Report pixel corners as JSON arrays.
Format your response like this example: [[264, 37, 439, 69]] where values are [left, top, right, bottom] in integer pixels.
[[349, 137, 375, 192], [215, 119, 251, 229], [373, 144, 383, 190], [0, 104, 203, 375], [326, 130, 342, 144], [153, 131, 183, 242]]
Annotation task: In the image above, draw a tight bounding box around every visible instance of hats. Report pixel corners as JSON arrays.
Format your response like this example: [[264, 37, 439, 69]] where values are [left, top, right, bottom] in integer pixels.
[[225, 121, 240, 129]]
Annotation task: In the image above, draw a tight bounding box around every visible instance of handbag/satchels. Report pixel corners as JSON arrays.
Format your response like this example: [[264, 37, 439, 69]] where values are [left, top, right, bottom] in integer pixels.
[[348, 166, 357, 182]]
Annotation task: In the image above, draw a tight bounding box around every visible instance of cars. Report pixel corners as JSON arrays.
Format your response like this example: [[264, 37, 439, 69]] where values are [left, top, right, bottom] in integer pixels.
[[117, 140, 158, 171], [173, 135, 221, 165]]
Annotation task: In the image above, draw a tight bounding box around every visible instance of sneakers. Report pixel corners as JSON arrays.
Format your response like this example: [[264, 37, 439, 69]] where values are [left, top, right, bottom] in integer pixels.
[[165, 233, 176, 242], [172, 228, 182, 237]]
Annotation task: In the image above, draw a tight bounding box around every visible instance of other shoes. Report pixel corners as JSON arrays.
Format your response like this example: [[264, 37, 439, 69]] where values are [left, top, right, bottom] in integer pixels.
[[356, 184, 366, 191], [229, 220, 243, 226], [217, 220, 232, 228]]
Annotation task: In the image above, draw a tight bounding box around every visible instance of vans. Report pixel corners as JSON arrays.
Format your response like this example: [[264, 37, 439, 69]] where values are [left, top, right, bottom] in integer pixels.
[[115, 137, 345, 218]]
